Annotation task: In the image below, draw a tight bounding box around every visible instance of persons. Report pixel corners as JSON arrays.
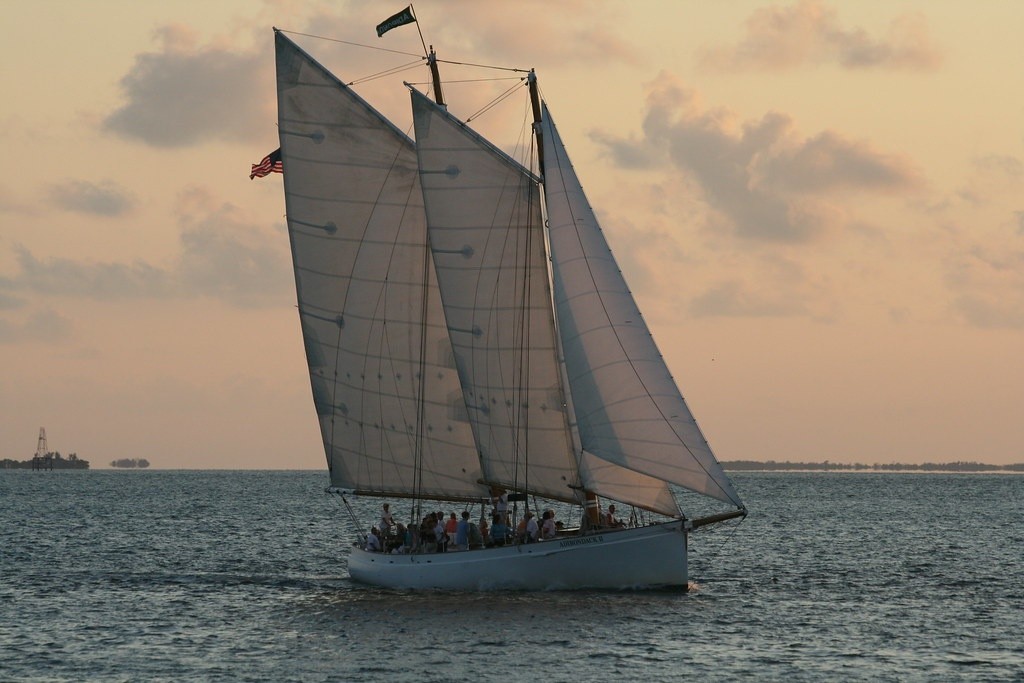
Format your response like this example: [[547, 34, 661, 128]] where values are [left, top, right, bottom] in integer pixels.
[[367, 503, 627, 556]]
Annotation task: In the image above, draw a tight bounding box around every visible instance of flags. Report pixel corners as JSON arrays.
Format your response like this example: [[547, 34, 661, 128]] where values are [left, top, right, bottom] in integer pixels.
[[376, 5, 417, 37], [249, 146, 284, 181]]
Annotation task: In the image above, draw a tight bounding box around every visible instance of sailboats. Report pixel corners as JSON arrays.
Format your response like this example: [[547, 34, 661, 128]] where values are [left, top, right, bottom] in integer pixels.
[[248, 2, 750, 597]]
